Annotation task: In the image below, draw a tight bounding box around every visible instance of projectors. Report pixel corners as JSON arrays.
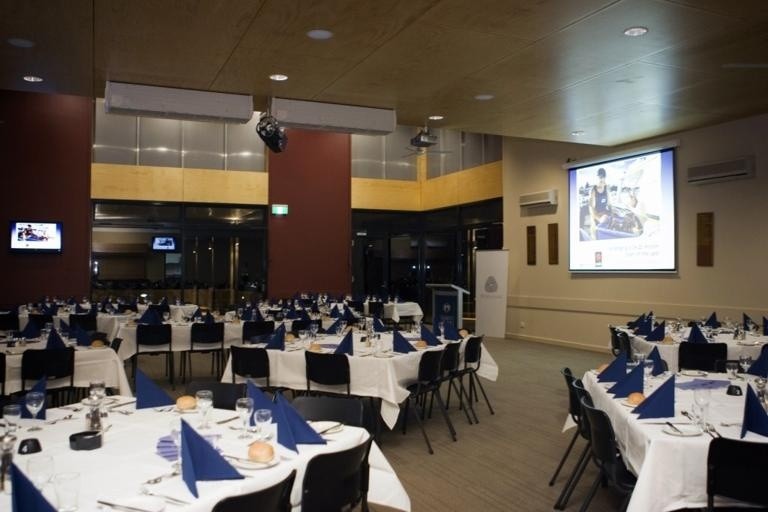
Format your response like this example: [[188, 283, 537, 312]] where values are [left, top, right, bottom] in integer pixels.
[[411, 134, 437, 147]]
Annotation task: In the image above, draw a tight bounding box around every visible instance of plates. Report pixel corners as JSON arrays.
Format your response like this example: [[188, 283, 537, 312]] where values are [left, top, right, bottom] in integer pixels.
[[232, 455, 282, 470], [308, 421, 344, 433], [98, 494, 164, 511], [661, 423, 702, 438], [590, 368, 639, 408], [373, 351, 392, 358]]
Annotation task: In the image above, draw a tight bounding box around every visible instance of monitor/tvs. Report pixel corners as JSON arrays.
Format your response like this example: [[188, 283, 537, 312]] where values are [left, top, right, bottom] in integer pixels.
[[8, 220, 63, 255], [152, 236, 179, 253]]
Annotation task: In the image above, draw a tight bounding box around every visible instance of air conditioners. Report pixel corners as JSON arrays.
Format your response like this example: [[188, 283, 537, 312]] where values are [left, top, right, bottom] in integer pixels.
[[683, 153, 756, 185], [518, 188, 557, 209]]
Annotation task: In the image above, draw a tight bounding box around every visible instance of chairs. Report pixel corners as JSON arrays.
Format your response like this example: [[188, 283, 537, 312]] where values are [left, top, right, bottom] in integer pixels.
[[546, 308, 767, 510], [3, 288, 501, 511]]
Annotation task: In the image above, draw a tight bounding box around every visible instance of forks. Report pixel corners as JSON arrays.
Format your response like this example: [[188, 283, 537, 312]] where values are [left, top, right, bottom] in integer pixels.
[[702, 423, 721, 439]]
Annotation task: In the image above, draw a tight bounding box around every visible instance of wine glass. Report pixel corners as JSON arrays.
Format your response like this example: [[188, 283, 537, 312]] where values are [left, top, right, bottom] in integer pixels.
[[646, 310, 759, 345], [2, 380, 275, 453], [632, 350, 768, 426]]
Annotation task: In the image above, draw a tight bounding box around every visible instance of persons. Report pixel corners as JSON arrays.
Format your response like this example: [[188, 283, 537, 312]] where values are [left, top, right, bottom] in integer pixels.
[[588, 168, 651, 240], [19, 224, 53, 243]]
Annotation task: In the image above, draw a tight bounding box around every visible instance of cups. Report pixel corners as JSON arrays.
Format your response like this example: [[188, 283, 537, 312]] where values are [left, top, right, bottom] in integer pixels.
[[298, 318, 424, 348], [25, 454, 79, 511], [5, 295, 123, 346], [143, 292, 399, 325]]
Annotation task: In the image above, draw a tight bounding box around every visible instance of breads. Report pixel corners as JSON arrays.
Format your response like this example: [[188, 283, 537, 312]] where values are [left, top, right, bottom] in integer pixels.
[[458, 329, 469, 337], [663, 336, 673, 345], [627, 392, 644, 404], [249, 443, 274, 464], [177, 395, 196, 411]]
